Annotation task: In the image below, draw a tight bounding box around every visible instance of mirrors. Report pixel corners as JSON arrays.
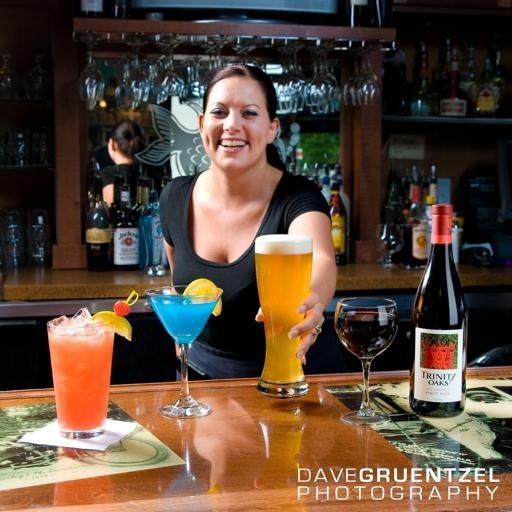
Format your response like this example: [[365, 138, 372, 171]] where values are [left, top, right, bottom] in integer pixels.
[[51, 17, 396, 272]]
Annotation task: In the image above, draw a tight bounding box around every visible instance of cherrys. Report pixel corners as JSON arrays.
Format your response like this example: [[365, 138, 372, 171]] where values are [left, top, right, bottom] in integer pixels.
[[114, 301, 131, 316]]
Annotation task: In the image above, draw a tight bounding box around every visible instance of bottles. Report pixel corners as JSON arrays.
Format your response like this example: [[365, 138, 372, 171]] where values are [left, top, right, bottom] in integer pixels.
[[408, 203, 472, 420], [383, 180, 439, 270], [328, 182, 348, 266], [406, 35, 508, 118], [5, 213, 49, 269], [110, 183, 142, 272], [141, 190, 168, 277], [84, 177, 114, 272]]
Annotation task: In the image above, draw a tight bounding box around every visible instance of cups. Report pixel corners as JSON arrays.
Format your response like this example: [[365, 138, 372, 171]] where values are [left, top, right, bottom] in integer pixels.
[[45, 315, 116, 440], [254, 233, 315, 399]]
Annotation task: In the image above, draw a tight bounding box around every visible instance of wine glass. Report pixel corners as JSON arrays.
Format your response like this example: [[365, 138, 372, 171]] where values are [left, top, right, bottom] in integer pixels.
[[77, 33, 384, 117], [144, 284, 213, 420], [334, 295, 400, 427]]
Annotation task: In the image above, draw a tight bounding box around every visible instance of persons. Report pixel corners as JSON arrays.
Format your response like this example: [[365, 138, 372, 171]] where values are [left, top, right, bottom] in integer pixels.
[[163, 387, 341, 512], [98, 118, 163, 211], [157, 62, 341, 386]]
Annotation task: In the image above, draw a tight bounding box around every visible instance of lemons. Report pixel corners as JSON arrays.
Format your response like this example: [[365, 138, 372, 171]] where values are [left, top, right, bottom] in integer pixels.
[[183, 279, 223, 316], [92, 311, 132, 341]]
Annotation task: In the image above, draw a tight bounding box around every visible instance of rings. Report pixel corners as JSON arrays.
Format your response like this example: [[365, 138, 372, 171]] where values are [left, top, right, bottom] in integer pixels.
[[313, 324, 323, 335]]
